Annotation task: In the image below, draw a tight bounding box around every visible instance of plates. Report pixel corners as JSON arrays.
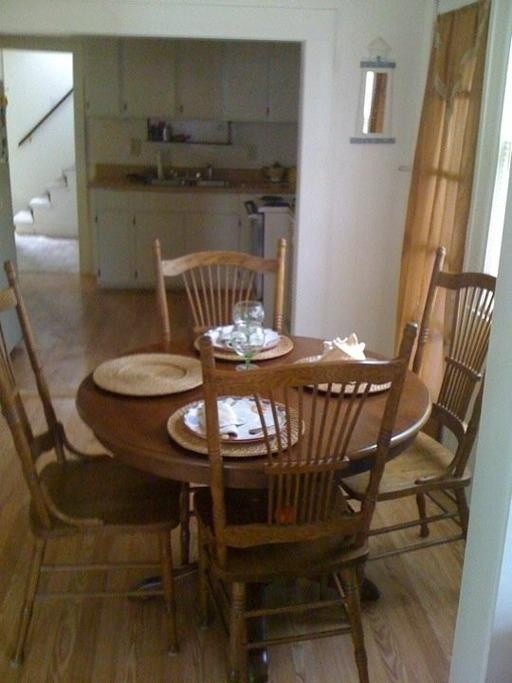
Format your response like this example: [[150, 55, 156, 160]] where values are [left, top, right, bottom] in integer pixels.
[[183, 394, 288, 443], [203, 321, 283, 355]]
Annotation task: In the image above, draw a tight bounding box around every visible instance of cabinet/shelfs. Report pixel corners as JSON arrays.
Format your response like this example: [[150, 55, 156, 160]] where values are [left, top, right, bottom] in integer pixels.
[[83, 36, 301, 120], [87, 193, 249, 293]]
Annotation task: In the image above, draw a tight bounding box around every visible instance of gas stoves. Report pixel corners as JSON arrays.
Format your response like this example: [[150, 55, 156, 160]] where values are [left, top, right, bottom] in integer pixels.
[[254, 195, 294, 213]]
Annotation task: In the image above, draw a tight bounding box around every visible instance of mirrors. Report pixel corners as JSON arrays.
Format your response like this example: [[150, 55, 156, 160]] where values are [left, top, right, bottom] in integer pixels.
[[349, 57, 397, 146]]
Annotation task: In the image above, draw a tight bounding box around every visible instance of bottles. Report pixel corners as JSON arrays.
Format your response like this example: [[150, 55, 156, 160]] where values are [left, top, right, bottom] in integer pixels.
[[155, 149, 166, 180]]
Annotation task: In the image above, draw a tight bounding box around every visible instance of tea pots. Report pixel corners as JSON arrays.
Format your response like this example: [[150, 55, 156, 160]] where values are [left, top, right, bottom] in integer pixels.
[[263, 159, 286, 183]]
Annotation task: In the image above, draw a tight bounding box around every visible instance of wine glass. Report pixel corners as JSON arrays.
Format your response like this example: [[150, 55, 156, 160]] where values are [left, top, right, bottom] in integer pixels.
[[233, 300, 265, 345], [229, 320, 265, 373]]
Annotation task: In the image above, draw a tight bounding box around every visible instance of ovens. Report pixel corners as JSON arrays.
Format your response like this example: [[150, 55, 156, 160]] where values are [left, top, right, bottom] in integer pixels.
[[240, 201, 293, 319]]
